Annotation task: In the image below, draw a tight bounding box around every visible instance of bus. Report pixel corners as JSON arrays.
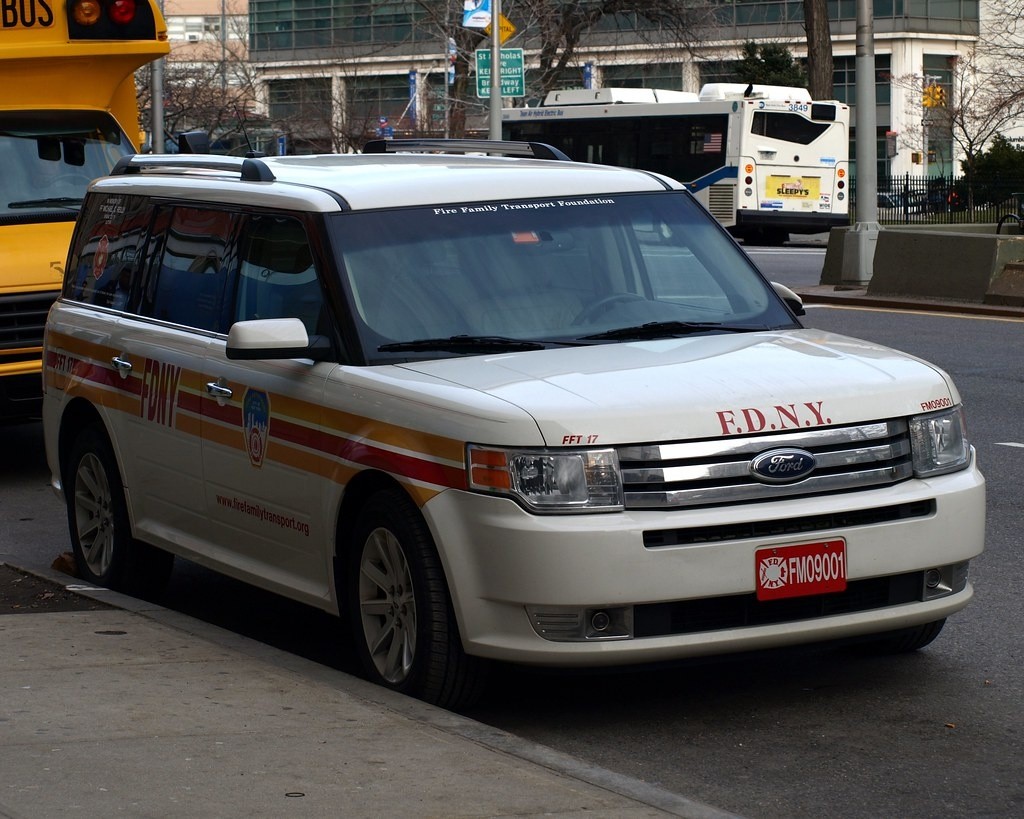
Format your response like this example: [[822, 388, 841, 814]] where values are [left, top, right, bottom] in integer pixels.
[[466, 84, 853, 247], [2, 0, 170, 469]]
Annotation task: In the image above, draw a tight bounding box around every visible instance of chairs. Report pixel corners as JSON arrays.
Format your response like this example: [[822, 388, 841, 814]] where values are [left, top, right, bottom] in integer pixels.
[[459, 232, 588, 336], [280, 226, 454, 349]]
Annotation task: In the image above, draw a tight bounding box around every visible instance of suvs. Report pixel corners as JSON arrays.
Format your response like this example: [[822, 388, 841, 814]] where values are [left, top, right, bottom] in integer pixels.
[[43, 137, 987, 710]]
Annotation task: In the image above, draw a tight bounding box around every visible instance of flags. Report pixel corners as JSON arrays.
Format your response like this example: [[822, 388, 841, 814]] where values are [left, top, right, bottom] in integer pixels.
[[703, 133, 722, 152]]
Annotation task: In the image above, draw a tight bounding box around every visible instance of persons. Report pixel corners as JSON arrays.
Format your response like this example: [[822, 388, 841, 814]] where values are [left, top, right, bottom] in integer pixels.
[[11, 140, 87, 205]]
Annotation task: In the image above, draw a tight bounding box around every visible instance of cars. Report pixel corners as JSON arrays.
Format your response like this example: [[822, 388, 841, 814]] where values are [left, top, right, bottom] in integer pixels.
[[875, 192, 918, 215]]
